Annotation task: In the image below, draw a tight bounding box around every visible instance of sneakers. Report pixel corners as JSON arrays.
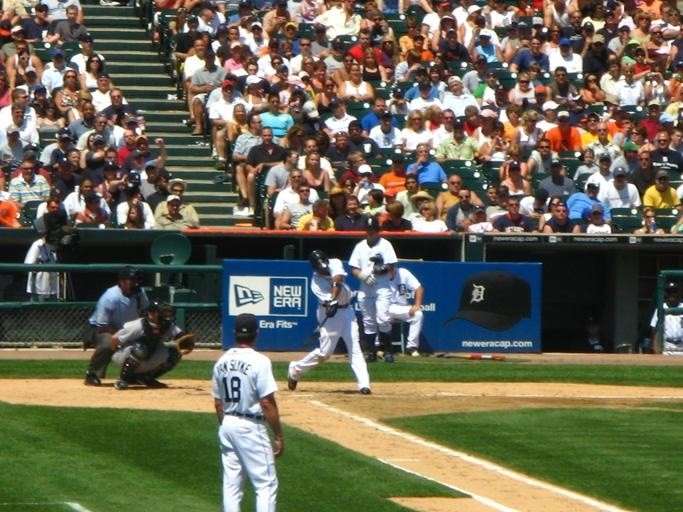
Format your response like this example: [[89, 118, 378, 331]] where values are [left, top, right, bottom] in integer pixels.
[[367, 348, 420, 363], [360, 387, 370, 394], [288, 361, 297, 389], [85, 370, 166, 390]]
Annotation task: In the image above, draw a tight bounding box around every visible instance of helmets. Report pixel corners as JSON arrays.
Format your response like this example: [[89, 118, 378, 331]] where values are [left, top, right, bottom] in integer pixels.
[[116, 266, 145, 296], [147, 298, 176, 333]]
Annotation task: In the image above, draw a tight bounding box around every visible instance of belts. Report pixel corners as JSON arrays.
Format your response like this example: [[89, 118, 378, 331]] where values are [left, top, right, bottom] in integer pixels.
[[225, 411, 261, 419]]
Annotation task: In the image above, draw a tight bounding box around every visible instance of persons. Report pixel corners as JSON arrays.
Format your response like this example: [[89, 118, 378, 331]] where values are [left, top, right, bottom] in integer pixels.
[[285, 246, 374, 397], [385, 263, 426, 359], [346, 215, 400, 364], [1, 0, 199, 231], [110, 300, 189, 391], [647, 280, 683, 356], [21, 227, 70, 304], [183, 0, 682, 244], [79, 263, 156, 389], [210, 311, 285, 512], [580, 322, 609, 353]]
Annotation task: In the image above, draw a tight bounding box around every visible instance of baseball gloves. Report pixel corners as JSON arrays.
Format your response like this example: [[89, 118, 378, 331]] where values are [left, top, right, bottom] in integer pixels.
[[175, 332, 194, 355]]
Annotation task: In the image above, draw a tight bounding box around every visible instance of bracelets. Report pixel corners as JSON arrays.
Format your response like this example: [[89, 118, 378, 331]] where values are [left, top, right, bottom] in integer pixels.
[[276, 431, 283, 437]]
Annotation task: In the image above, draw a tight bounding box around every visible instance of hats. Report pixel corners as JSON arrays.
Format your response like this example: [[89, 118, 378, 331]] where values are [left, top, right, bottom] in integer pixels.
[[365, 217, 378, 230], [11, 4, 186, 205], [446, 272, 531, 330], [309, 249, 329, 269], [177, 0, 682, 214], [235, 314, 256, 332], [666, 282, 679, 292]]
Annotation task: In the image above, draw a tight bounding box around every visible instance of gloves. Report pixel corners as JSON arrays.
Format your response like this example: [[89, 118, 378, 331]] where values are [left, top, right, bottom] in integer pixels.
[[326, 299, 338, 316], [359, 272, 376, 286]]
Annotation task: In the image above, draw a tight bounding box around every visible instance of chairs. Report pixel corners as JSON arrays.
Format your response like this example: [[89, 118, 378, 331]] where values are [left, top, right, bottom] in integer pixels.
[[0, 0, 681, 241]]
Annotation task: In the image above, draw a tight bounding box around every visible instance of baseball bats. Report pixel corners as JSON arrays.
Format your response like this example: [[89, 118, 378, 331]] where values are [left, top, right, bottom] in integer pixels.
[[313, 316, 329, 333]]
